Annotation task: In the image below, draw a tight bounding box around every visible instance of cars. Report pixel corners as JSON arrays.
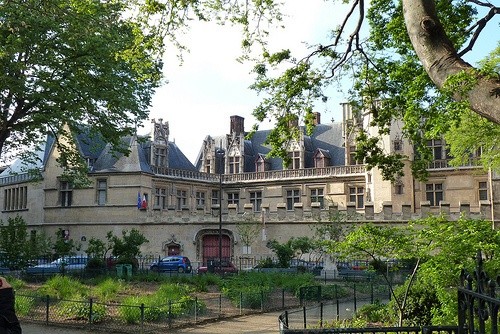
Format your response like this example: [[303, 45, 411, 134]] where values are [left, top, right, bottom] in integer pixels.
[[150, 255, 192, 273], [35, 254, 92, 270], [196, 260, 238, 276], [242, 262, 282, 273]]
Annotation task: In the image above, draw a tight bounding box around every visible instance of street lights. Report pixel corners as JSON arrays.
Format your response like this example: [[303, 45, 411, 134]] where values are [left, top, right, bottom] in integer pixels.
[[217, 140, 225, 274]]
